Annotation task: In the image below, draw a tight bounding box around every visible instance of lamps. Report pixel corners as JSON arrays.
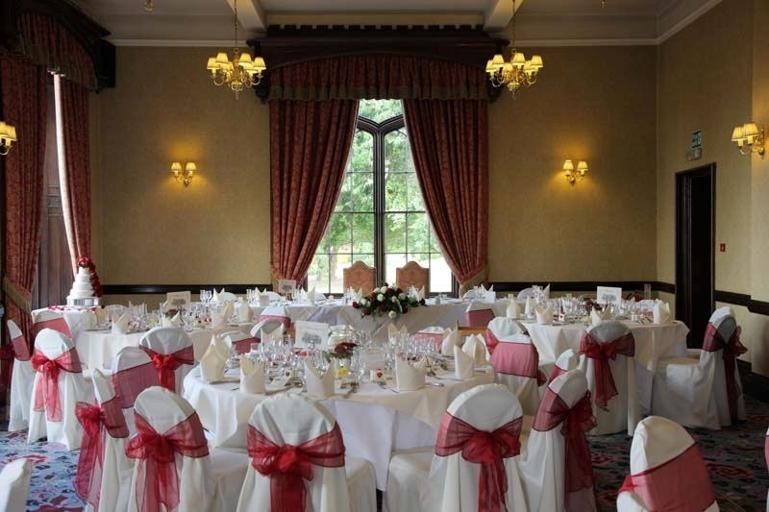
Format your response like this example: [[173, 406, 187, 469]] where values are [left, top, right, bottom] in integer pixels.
[[169, 161, 198, 187], [206, 0, 266, 101], [563, 159, 589, 184], [485, 1, 544, 101], [0, 121, 18, 155], [729, 120, 766, 158]]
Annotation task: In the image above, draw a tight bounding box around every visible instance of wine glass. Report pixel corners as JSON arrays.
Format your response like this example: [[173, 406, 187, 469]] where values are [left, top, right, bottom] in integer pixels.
[[506, 293, 654, 325], [211, 328, 440, 393], [107, 285, 356, 329]]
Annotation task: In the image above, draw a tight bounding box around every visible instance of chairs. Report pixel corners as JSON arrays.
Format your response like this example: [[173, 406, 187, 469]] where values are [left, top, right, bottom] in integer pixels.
[[658, 305, 744, 428], [1, 457, 31, 511], [75, 366, 134, 512], [380, 383, 526, 512], [132, 386, 248, 511], [235, 390, 378, 511], [501, 370, 599, 511]]
